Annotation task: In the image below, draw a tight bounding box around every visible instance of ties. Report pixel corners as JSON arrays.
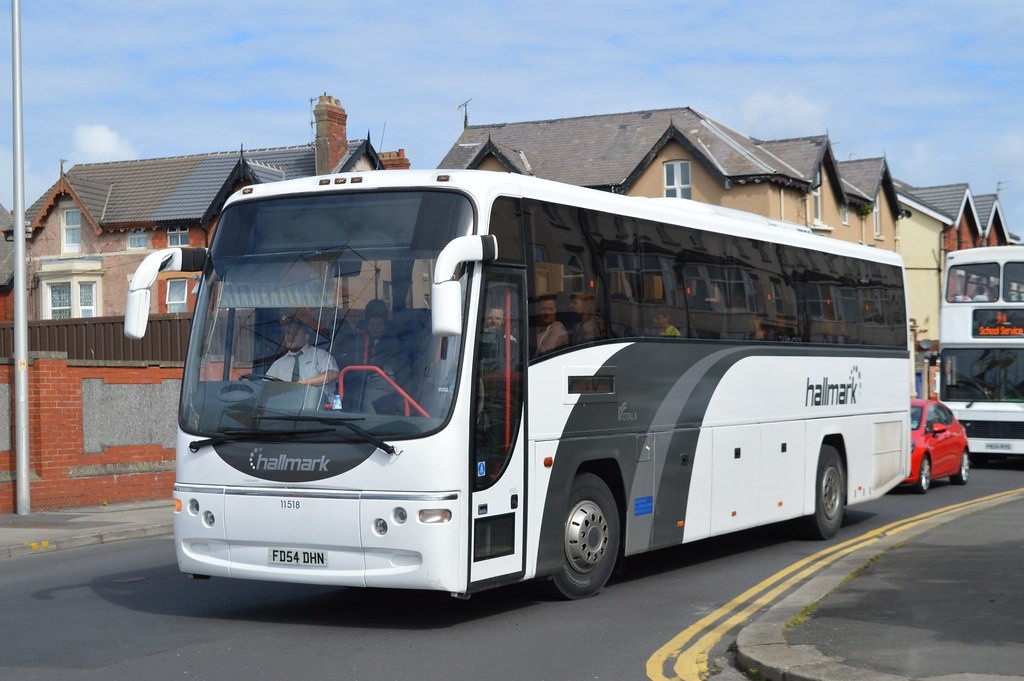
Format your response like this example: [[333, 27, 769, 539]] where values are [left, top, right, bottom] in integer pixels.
[[292, 351, 304, 383]]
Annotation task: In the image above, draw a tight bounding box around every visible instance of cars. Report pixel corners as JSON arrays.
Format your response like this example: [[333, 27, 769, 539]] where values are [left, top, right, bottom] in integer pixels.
[[901, 399, 970, 493]]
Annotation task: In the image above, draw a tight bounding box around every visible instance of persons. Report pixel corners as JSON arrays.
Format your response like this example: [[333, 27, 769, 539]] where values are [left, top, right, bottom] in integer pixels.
[[261, 321, 340, 406], [358, 298, 850, 414]]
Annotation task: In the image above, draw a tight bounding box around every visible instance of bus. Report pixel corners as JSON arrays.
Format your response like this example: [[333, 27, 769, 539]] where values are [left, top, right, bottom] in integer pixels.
[[936, 245, 1024, 467], [125, 168, 914, 602]]
